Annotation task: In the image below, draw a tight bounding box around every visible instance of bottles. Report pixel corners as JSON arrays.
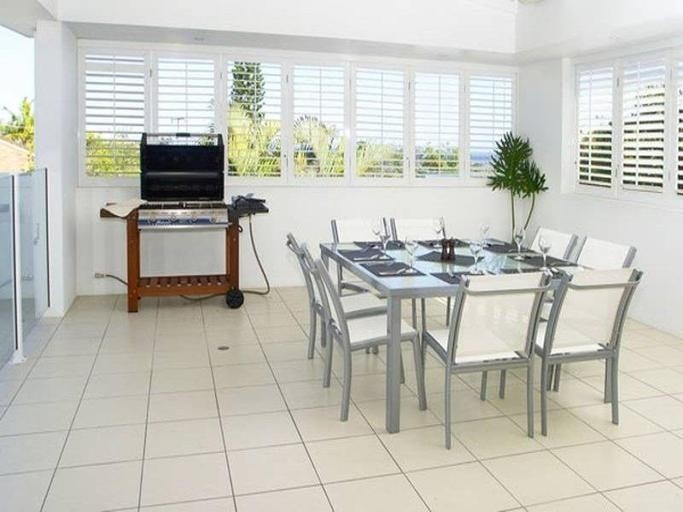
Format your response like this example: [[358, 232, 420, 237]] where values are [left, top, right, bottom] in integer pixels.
[[441, 239, 455, 260]]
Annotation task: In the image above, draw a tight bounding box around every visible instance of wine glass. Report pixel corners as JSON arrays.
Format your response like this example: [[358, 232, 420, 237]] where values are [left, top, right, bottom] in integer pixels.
[[432, 217, 444, 247], [469, 240, 483, 272], [539, 234, 552, 270], [404, 236, 419, 274], [512, 226, 526, 261], [480, 223, 490, 248], [380, 226, 392, 259], [370, 216, 383, 249]]
